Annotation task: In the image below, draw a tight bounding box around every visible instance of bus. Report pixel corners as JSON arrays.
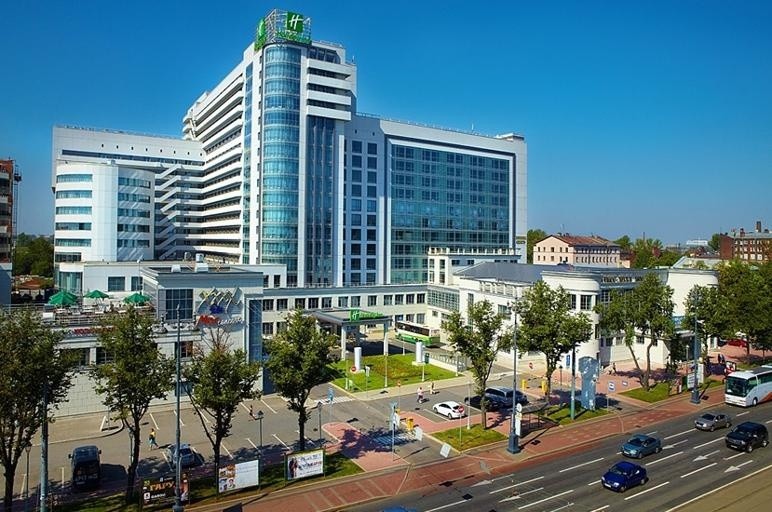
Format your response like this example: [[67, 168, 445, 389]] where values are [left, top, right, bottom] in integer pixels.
[[720, 363, 771, 407]]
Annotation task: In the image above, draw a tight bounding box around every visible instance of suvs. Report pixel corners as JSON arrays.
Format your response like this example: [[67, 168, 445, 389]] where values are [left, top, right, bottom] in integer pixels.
[[163, 441, 196, 467], [723, 419, 769, 453]]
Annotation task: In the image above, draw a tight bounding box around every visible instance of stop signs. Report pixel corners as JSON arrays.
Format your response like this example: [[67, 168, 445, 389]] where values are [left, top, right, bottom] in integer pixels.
[[457, 404, 465, 413]]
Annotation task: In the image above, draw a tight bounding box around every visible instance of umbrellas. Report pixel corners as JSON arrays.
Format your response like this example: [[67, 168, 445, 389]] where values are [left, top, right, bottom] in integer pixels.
[[122, 293, 150, 304], [83, 290, 109, 304], [47, 289, 78, 307]]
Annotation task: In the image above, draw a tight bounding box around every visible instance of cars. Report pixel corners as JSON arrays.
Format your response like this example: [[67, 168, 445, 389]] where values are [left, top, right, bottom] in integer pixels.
[[599, 458, 649, 493], [463, 385, 529, 412], [433, 400, 466, 419], [717, 331, 751, 347], [693, 411, 732, 432], [620, 434, 663, 459]]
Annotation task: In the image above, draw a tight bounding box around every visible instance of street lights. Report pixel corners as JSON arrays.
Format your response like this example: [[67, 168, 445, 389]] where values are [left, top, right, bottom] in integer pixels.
[[504, 290, 532, 453], [315, 399, 323, 453], [688, 289, 707, 403], [255, 408, 266, 475], [23, 437, 34, 511], [559, 363, 564, 411], [152, 303, 208, 512]]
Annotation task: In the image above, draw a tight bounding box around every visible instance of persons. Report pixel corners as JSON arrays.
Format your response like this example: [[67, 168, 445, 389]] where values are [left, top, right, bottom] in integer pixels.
[[248, 405, 256, 421], [149, 428, 159, 451], [416, 387, 424, 404], [223, 484, 227, 490], [109, 302, 114, 312], [428, 379, 436, 395], [229, 479, 235, 489], [690, 362, 695, 372], [119, 301, 121, 307], [528, 363, 536, 379], [613, 363, 616, 376]]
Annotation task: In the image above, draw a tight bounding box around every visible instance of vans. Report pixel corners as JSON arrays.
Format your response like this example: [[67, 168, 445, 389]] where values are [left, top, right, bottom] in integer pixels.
[[65, 443, 105, 489]]
